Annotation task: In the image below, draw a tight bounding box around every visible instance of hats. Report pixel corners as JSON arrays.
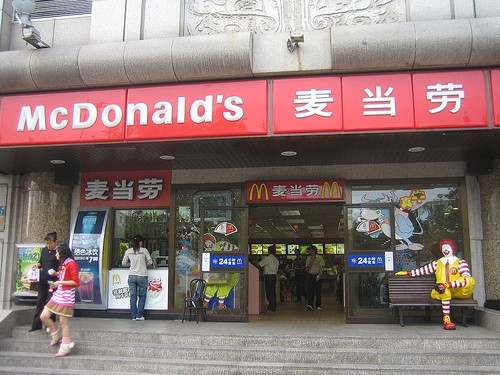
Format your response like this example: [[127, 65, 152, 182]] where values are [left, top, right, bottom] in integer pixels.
[[307, 246, 318, 254]]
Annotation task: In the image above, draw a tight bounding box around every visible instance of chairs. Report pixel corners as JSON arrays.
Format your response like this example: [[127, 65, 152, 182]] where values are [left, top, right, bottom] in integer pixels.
[[182, 278, 207, 324]]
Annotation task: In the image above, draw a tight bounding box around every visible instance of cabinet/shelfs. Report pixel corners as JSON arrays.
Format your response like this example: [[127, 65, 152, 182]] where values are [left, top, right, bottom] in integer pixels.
[[249, 262, 265, 314]]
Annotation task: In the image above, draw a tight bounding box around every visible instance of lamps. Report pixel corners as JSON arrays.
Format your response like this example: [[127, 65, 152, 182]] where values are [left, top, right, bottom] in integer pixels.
[[287, 32, 304, 53], [12, 0, 51, 48]]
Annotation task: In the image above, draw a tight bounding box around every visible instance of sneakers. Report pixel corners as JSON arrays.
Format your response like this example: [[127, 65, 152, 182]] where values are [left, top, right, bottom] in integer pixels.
[[49, 329, 63, 346], [132, 318, 136, 320], [136, 316, 145, 321], [54, 341, 76, 356]]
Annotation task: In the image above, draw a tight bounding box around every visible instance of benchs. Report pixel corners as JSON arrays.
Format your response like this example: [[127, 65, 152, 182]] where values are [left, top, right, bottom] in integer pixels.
[[387, 276, 478, 327]]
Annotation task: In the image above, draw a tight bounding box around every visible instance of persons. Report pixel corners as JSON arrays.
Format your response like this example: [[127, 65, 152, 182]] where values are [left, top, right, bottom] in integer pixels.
[[277, 259, 298, 300], [249, 254, 266, 267], [289, 250, 309, 303], [395, 237, 476, 330], [256, 246, 279, 313], [305, 246, 325, 311], [122, 234, 153, 321], [29, 231, 59, 333], [334, 272, 343, 298], [40, 243, 81, 357], [325, 258, 343, 280]]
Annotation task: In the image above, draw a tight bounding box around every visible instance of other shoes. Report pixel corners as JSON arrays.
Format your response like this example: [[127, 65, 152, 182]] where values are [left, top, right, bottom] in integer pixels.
[[316, 306, 323, 310], [28, 326, 43, 332], [305, 304, 314, 310]]
